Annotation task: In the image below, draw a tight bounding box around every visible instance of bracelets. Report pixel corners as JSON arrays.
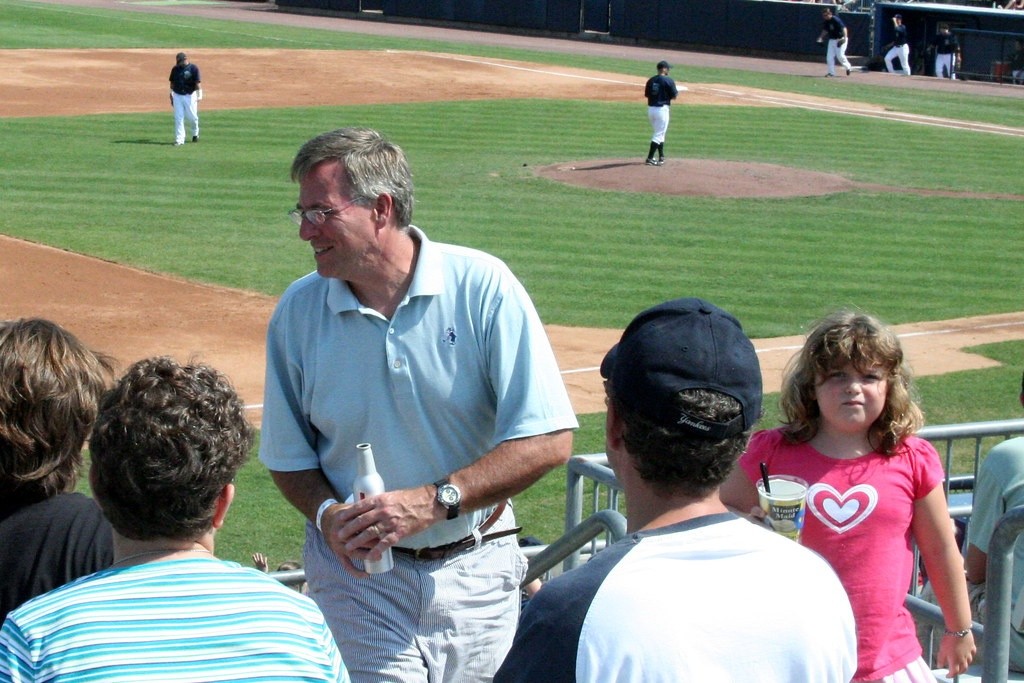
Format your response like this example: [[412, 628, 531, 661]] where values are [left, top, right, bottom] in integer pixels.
[[945, 624, 972, 639], [316, 498, 338, 532]]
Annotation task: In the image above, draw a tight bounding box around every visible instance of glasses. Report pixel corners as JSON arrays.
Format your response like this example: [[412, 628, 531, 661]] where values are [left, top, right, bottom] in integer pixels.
[[287, 193, 369, 225]]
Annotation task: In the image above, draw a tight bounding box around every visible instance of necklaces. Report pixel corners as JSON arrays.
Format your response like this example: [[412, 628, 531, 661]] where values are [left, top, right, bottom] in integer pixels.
[[109, 548, 210, 568]]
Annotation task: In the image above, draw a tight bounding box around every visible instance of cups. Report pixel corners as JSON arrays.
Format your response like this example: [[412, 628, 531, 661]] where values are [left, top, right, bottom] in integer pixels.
[[757, 475, 807, 545]]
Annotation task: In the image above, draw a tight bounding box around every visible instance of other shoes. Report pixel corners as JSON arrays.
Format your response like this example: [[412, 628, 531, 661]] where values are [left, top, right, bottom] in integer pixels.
[[174, 142, 181, 145], [193, 135, 199, 142], [645, 158, 656, 165], [846, 69, 851, 76], [825, 72, 834, 78], [657, 157, 665, 165]]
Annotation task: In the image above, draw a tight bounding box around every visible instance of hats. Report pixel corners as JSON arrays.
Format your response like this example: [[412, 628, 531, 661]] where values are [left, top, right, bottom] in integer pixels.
[[893, 14, 902, 18], [657, 61, 673, 70], [176, 53, 186, 66], [599, 297, 763, 439]]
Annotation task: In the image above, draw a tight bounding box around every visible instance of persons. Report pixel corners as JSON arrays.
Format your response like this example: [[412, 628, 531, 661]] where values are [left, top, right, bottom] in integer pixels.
[[1011, 42, 1024, 84], [169, 52, 202, 146], [884, 14, 911, 75], [818, 8, 852, 77], [0, 318, 354, 683], [491, 298, 1024, 683], [761, 0, 1024, 12], [928, 23, 962, 78], [257, 126, 580, 683], [645, 61, 678, 166]]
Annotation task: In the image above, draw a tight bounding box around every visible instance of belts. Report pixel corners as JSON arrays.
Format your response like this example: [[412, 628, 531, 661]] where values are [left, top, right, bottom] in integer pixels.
[[391, 497, 523, 560], [895, 45, 903, 47]]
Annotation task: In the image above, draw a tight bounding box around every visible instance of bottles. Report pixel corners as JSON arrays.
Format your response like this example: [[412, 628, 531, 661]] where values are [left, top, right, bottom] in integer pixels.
[[354, 442, 394, 574]]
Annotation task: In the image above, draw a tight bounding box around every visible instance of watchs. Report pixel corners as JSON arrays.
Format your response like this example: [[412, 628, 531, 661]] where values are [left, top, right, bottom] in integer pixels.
[[433, 478, 461, 520]]
